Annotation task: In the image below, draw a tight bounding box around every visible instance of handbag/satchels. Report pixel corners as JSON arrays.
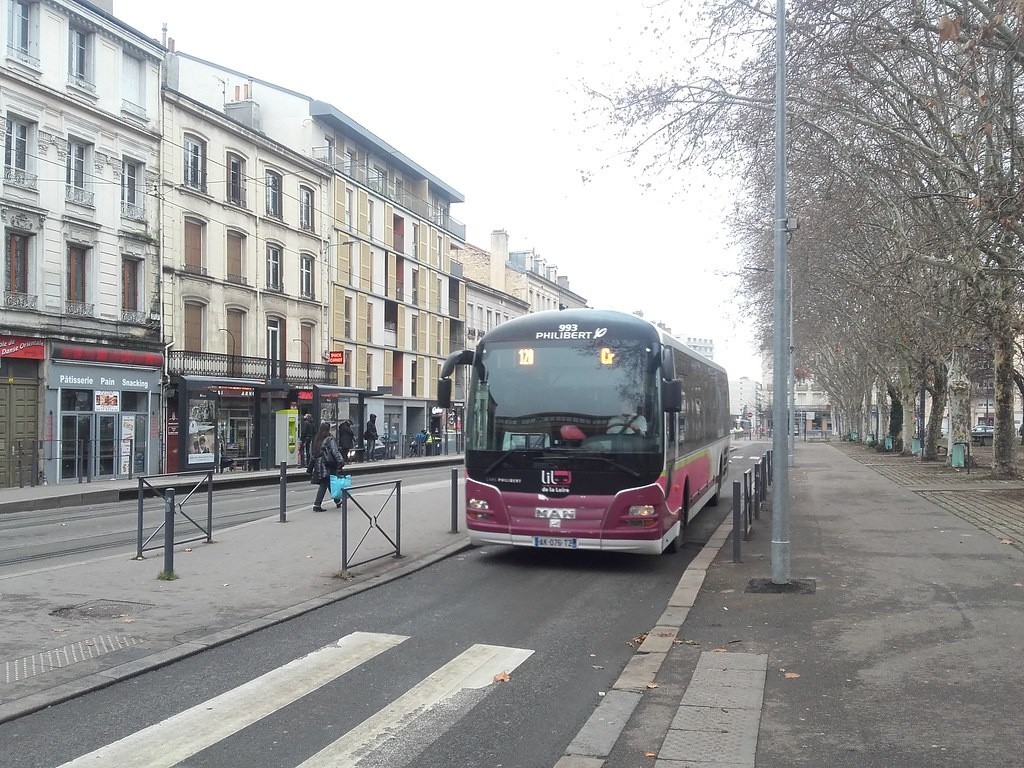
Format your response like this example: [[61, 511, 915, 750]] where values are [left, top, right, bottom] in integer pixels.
[[329, 467, 352, 500]]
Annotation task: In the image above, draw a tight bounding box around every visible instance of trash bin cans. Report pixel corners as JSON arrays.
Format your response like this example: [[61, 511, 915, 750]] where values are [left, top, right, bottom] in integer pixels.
[[425, 442, 432, 456], [434, 439, 442, 455]]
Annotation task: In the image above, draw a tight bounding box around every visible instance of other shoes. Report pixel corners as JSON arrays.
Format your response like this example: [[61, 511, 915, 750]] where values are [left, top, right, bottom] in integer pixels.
[[337, 502, 342, 508], [312, 505, 327, 513], [344, 461, 351, 465], [367, 458, 378, 462]]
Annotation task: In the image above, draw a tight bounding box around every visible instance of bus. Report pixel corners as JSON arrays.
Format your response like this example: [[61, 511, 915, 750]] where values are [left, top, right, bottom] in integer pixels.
[[438, 308, 731, 555]]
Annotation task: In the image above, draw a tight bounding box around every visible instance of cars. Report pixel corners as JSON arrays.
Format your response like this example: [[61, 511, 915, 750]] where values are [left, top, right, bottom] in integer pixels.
[[971, 426, 994, 434]]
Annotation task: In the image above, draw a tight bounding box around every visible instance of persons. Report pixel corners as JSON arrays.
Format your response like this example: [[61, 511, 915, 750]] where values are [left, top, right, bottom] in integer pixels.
[[301, 413, 381, 512], [606, 398, 647, 436]]
